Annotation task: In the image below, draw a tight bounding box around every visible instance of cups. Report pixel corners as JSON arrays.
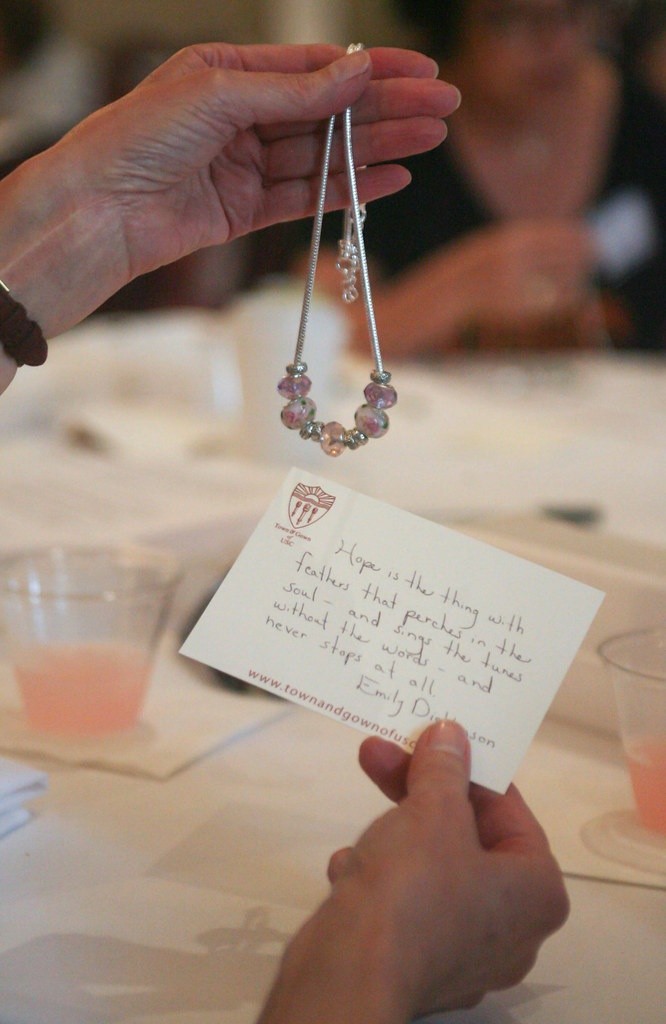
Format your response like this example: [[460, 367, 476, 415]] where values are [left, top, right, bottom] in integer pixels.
[[598, 628, 666, 828], [0, 548, 183, 731]]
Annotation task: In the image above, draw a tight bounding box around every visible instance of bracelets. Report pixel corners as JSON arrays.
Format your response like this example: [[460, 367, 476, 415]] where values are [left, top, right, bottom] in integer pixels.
[[0, 281, 48, 367]]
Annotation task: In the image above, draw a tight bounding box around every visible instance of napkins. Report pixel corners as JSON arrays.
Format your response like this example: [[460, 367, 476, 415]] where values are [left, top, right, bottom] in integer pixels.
[[2, 683, 294, 783], [551, 785, 662, 888]]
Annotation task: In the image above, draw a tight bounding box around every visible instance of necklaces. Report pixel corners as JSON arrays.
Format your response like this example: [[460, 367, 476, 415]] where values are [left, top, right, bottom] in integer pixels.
[[279, 43, 398, 456]]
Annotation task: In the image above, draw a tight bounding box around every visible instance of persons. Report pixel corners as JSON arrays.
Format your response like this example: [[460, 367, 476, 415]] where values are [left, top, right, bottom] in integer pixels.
[[0, 0, 666, 363], [0, 43, 568, 1024]]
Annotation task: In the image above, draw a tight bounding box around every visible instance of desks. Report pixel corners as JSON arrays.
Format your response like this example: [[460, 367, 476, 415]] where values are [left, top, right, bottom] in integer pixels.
[[0, 305, 666, 1024]]
